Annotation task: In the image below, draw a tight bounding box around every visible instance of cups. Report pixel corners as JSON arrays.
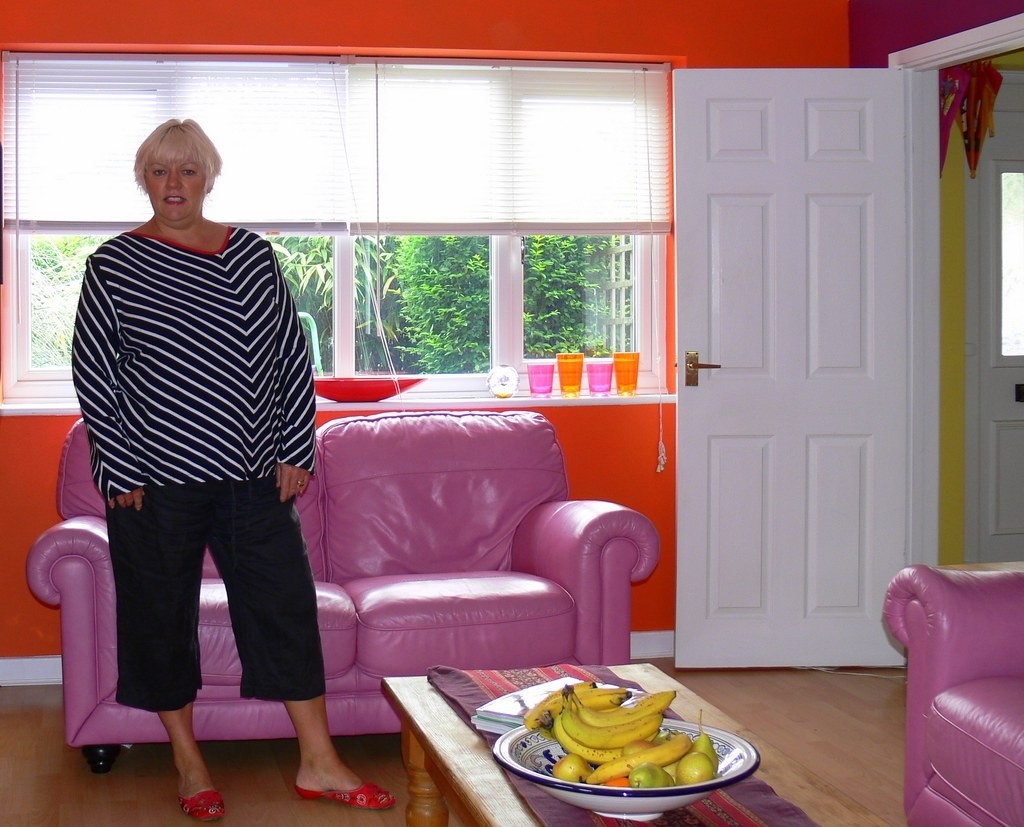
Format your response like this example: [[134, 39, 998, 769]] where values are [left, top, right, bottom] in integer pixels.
[[556, 353, 584, 398], [613, 352, 640, 395], [527, 362, 555, 398], [584, 358, 613, 396]]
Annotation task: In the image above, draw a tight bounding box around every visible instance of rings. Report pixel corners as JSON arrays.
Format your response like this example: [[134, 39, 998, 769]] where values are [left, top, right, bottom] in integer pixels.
[[296, 480, 305, 487]]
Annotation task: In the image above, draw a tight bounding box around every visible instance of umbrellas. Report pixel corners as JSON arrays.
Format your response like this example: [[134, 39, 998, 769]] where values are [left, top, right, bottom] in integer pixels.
[[939, 66, 971, 179], [954, 59, 1004, 179]]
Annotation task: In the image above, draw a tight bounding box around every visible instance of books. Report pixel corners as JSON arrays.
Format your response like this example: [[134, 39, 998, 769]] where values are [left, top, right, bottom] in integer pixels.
[[470, 676, 653, 735]]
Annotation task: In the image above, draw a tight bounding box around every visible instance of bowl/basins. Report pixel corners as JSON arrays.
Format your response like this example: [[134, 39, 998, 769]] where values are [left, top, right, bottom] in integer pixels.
[[313, 378, 427, 402], [493, 718, 761, 821]]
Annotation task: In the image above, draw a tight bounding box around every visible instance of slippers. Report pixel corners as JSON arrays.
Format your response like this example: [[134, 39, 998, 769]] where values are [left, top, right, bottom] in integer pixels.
[[176, 777, 226, 821], [295, 783, 396, 811]]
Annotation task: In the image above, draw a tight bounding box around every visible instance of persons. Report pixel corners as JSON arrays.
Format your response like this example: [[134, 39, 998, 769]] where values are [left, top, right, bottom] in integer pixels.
[[70, 118, 396, 823]]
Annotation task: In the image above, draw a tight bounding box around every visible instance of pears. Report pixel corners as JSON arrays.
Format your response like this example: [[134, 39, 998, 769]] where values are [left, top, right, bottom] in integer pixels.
[[551, 708, 719, 789]]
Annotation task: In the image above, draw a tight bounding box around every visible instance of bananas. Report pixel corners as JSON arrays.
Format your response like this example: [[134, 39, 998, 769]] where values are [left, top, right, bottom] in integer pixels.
[[522, 682, 694, 783]]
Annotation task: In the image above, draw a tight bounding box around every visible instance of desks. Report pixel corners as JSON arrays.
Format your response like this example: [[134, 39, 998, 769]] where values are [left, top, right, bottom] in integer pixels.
[[380, 663, 896, 827]]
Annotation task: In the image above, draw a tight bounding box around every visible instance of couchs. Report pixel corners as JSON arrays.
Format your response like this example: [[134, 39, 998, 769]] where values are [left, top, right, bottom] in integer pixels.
[[884, 560, 1024, 827], [26, 411, 660, 773]]
